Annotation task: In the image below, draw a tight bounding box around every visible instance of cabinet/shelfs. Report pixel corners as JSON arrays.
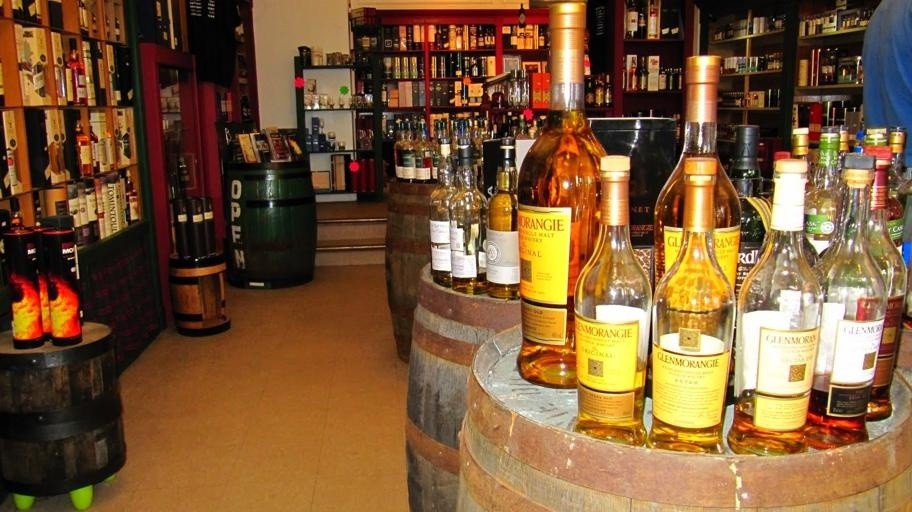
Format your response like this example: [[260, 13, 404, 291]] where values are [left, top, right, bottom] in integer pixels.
[[294, 50, 358, 202], [348, 6, 549, 194]]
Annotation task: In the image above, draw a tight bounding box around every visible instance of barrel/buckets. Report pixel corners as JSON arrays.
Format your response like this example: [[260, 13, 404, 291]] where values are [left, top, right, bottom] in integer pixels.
[[222, 161, 317, 290], [403, 261, 520, 511], [453, 323, 912, 511], [384, 182, 436, 364]]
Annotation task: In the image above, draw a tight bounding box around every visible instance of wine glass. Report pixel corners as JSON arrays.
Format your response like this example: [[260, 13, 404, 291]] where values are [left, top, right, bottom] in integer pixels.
[[357, 129, 374, 150], [304, 79, 373, 109], [381, 81, 388, 107]]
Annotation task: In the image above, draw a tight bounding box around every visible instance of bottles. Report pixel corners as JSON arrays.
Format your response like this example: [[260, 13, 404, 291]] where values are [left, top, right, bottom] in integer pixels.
[[297, 46, 323, 66], [88, 111, 100, 174], [125, 169, 139, 223], [76, 109, 93, 178], [9, 197, 25, 227], [92, 11, 121, 41], [64, 37, 89, 107], [179, 157, 191, 185], [393, 0, 911, 457]]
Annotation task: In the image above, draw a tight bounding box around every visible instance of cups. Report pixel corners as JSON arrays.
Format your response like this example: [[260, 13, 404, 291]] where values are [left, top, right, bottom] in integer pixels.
[[319, 118, 345, 150]]
